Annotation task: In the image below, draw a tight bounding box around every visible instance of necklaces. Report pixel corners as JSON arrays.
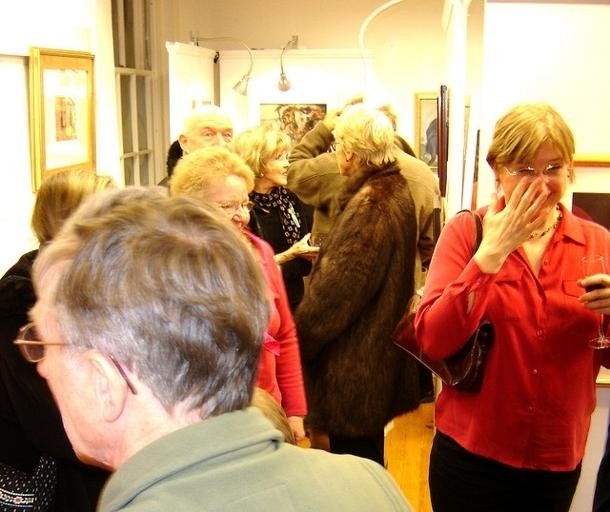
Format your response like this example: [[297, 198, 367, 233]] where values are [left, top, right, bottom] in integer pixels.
[[524, 204, 562, 241]]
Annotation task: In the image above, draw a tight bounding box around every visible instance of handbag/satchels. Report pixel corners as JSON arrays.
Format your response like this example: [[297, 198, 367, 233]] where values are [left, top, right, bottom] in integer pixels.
[[390, 287, 496, 395], [0, 453, 62, 511]]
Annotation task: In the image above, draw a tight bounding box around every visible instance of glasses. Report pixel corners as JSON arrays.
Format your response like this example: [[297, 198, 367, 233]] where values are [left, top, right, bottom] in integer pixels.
[[13, 323, 138, 399], [502, 160, 567, 184], [332, 140, 340, 150], [214, 200, 253, 216]]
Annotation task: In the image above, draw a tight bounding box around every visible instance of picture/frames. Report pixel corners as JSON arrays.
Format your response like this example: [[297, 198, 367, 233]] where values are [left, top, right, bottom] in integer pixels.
[[414, 92, 440, 176], [28, 45, 97, 194]]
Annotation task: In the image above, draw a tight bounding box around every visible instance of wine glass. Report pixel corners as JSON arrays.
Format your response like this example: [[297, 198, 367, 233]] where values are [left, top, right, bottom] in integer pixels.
[[581, 254, 610, 350], [303, 232, 327, 279]]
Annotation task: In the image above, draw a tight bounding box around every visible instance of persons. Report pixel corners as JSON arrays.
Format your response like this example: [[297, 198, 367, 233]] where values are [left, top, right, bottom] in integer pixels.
[[3, 93, 447, 512], [410, 101, 610, 512]]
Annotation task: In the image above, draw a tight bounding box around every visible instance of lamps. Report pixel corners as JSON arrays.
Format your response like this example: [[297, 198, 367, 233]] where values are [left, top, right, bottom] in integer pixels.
[[189, 29, 301, 97]]
